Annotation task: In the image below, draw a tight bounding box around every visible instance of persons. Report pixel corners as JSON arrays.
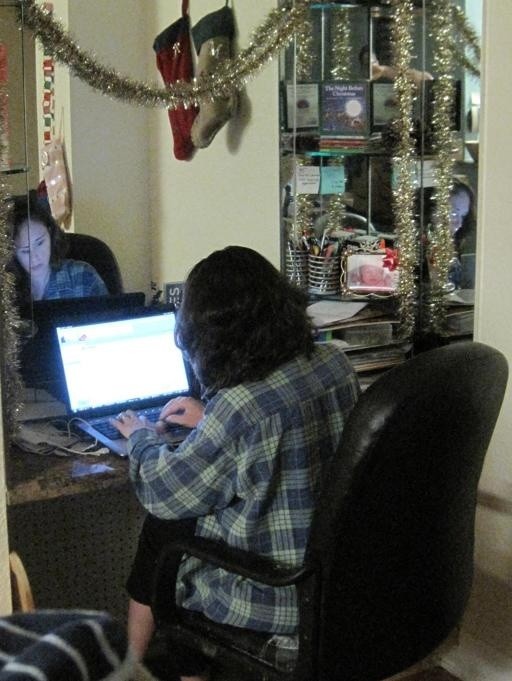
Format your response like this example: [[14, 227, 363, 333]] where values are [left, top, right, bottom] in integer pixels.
[[5, 195, 109, 304], [109, 247, 366, 679], [420, 171, 476, 288]]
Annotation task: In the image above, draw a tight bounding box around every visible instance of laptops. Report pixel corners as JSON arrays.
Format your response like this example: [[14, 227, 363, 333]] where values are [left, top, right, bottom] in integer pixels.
[[28, 291, 145, 381], [52, 300, 202, 458]]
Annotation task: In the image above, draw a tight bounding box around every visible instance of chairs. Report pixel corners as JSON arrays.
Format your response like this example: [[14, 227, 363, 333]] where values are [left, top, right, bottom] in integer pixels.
[[148, 342, 510, 681], [49, 234, 123, 301]]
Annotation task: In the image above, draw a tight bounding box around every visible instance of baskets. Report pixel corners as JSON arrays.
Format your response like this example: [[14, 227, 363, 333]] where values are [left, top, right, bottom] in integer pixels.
[[307, 253, 340, 295], [284, 249, 308, 289]]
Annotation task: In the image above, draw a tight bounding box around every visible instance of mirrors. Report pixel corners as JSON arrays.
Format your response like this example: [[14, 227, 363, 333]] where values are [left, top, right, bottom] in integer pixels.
[[0, 2, 284, 407]]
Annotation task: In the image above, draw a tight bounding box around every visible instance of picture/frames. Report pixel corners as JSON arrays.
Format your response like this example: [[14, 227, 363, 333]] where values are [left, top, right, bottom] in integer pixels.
[[280, 76, 401, 142]]
